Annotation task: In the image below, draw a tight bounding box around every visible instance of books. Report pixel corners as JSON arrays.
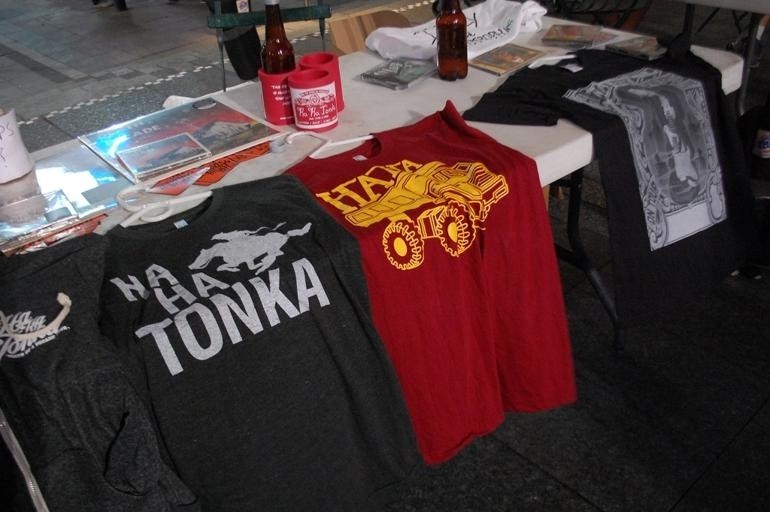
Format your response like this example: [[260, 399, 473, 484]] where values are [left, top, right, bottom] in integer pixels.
[[467, 44, 544, 76], [359, 56, 436, 92], [117, 133, 214, 178], [543, 23, 604, 47]]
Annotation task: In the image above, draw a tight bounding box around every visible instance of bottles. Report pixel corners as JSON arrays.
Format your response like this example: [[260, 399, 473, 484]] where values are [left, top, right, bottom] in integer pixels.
[[262, 0, 296, 74], [436, 0, 468, 81]]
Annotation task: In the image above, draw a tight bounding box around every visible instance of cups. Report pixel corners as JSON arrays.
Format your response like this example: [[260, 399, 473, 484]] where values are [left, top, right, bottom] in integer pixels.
[[258, 65, 300, 125], [287, 69, 338, 133], [299, 52, 344, 113]]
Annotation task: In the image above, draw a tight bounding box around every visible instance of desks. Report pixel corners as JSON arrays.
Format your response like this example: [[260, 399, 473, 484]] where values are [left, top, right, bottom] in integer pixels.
[[681, 0, 770, 116], [1, 10, 744, 441]]
